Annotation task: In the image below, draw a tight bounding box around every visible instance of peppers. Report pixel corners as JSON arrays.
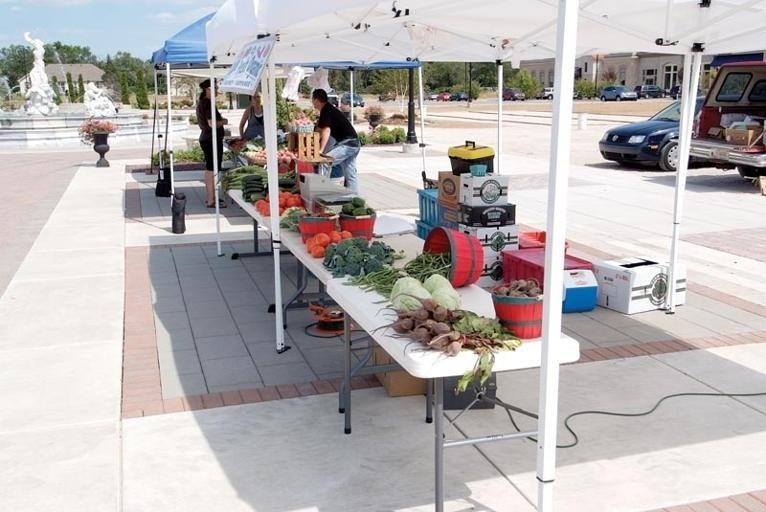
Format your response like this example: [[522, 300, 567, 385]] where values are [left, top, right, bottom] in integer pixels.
[[343, 197, 375, 216]]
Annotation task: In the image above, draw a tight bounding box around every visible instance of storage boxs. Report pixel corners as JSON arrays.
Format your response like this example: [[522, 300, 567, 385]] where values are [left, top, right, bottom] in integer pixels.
[[726, 127, 762, 145], [372, 338, 435, 397], [415, 173, 686, 315]]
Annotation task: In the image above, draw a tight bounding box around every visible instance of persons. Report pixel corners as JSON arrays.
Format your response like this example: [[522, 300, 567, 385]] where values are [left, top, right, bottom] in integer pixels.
[[196, 78, 228, 209], [312, 88, 362, 194], [238, 90, 264, 139]]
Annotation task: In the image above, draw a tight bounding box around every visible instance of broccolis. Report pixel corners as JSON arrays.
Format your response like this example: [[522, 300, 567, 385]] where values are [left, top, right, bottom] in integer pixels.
[[324, 236, 406, 276]]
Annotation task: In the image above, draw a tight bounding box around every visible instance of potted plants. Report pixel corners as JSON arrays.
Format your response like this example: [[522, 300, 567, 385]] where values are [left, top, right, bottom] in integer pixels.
[[364, 106, 385, 128], [340, 104, 349, 117]]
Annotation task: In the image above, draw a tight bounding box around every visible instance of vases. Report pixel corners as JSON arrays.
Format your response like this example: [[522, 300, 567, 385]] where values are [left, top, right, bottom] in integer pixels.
[[93, 133, 110, 167]]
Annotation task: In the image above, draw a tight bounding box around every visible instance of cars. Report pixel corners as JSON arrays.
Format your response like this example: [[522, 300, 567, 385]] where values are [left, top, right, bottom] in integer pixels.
[[425, 91, 469, 101], [325, 88, 339, 107]]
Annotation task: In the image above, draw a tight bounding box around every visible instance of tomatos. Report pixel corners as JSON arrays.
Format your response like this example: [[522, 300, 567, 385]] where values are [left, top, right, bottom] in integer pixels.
[[306, 230, 352, 258], [255, 190, 303, 216]]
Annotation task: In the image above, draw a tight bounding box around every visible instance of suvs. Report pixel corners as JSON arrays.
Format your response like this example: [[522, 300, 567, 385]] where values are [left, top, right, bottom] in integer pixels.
[[633, 84, 666, 99], [502, 87, 525, 101], [597, 94, 742, 171], [540, 87, 554, 100], [599, 85, 637, 101], [669, 85, 702, 99], [340, 92, 364, 107]]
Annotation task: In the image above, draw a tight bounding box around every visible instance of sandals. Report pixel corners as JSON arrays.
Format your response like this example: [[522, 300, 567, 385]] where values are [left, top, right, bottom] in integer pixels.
[[205, 198, 228, 209]]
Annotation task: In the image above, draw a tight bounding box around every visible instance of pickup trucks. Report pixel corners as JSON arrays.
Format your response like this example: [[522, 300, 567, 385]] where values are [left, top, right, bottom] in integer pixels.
[[688, 59, 766, 181]]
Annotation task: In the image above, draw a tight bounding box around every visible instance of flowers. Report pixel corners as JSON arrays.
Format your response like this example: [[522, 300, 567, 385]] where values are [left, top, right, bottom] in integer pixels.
[[79, 117, 116, 144]]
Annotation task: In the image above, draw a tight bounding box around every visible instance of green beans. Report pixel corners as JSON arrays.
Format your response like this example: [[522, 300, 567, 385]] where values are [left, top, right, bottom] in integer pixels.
[[342, 247, 451, 303]]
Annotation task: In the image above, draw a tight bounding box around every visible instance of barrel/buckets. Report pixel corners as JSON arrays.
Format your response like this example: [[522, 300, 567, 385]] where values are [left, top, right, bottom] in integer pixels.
[[297, 213, 340, 243], [423, 226, 484, 289], [338, 211, 376, 241], [288, 159, 314, 184], [490, 284, 543, 339]]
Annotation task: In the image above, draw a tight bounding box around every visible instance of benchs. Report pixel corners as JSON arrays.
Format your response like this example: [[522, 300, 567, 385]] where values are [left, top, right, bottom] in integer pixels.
[[182, 136, 199, 148]]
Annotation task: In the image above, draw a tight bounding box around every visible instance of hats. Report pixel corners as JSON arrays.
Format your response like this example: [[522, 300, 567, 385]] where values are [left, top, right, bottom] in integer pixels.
[[199, 78, 217, 89]]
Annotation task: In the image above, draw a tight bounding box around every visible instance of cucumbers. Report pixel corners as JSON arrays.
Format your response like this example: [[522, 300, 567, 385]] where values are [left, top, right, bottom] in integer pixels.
[[242, 174, 301, 202]]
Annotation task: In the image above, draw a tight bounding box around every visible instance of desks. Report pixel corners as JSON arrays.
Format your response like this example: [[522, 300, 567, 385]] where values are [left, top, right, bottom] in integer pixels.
[[228, 190, 425, 413], [223, 141, 266, 166], [328, 278, 580, 512]]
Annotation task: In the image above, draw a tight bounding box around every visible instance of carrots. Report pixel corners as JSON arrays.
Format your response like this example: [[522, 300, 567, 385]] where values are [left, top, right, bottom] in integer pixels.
[[497, 278, 541, 298], [391, 294, 461, 355]]
